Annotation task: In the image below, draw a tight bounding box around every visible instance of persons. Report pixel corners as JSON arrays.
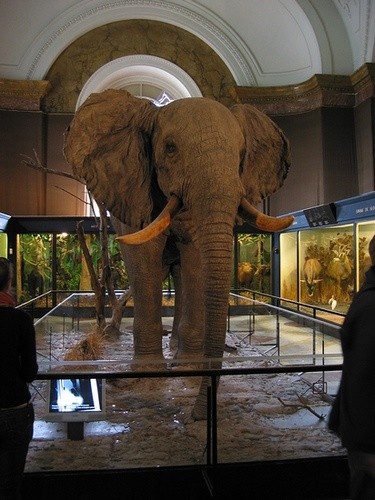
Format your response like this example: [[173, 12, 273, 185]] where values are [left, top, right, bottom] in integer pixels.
[[0, 257, 39, 473], [326, 236, 375, 500]]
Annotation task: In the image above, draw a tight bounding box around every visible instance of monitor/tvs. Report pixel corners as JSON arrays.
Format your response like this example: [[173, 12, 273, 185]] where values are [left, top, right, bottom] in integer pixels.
[[47, 378, 106, 422]]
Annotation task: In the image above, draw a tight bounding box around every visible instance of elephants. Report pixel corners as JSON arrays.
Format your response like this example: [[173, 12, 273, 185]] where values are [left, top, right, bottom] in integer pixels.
[[62, 87, 296, 422]]
